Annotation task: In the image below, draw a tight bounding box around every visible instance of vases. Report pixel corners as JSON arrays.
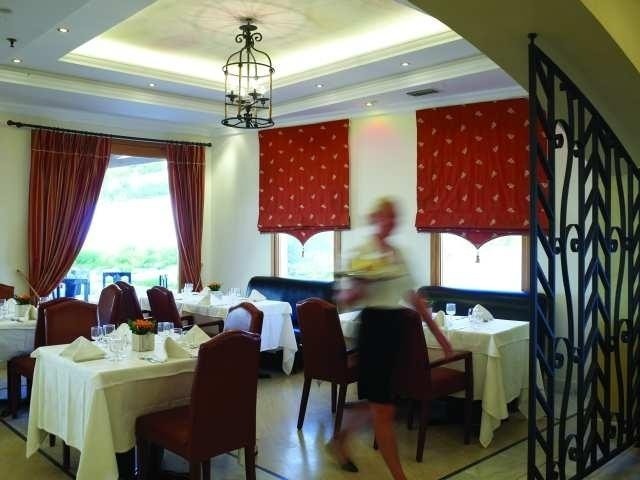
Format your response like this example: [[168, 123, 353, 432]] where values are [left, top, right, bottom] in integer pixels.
[[15, 304, 30, 316], [208, 291, 223, 299], [130, 332, 155, 351]]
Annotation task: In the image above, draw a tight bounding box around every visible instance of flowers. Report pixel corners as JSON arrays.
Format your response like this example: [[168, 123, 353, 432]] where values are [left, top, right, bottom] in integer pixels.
[[15, 292, 31, 304], [207, 282, 222, 292], [127, 317, 158, 334]]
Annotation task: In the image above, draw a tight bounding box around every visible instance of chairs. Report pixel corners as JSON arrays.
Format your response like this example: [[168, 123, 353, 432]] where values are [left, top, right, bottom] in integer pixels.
[[222, 303, 264, 337], [43, 300, 98, 345], [6, 298, 81, 419], [98, 285, 123, 328], [370, 310, 473, 462], [114, 281, 152, 326], [53, 279, 90, 301], [144, 286, 224, 339], [134, 331, 262, 480], [0, 283, 15, 300], [292, 298, 360, 439]]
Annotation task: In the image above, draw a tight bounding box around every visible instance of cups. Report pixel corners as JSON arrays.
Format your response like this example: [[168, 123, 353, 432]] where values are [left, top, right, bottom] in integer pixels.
[[170, 328, 182, 340]]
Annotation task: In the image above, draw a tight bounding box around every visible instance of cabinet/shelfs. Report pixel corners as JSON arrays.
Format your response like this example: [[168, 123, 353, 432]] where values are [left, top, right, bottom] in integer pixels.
[[103, 273, 132, 285]]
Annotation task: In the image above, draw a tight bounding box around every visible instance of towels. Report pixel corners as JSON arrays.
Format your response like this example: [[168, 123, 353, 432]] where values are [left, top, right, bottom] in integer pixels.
[[248, 288, 267, 301], [196, 294, 219, 306], [472, 304, 495, 321], [59, 336, 106, 362], [184, 324, 212, 346], [152, 337, 191, 362], [19, 306, 39, 321]]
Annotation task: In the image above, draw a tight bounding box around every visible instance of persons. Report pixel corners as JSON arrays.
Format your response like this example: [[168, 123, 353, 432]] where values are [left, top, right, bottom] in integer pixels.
[[326, 196, 453, 479]]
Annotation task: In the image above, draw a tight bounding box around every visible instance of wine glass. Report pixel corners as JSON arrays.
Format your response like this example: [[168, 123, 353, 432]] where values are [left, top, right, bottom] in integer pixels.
[[1, 304, 7, 320], [110, 333, 122, 364], [103, 324, 115, 349], [446, 304, 456, 323], [91, 327, 102, 346], [0, 299, 6, 308], [37, 297, 48, 307], [158, 322, 174, 346]]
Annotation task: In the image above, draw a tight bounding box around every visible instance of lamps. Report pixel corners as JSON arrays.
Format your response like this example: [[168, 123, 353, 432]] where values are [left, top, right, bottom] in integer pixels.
[[221, 17, 276, 130]]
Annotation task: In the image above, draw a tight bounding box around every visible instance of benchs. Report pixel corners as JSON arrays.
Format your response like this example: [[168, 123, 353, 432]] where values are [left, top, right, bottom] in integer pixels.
[[414, 286, 554, 378], [243, 277, 336, 379]]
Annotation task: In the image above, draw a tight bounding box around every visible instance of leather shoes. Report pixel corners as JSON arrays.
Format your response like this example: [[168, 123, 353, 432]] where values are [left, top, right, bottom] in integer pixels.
[[326, 442, 359, 473]]
